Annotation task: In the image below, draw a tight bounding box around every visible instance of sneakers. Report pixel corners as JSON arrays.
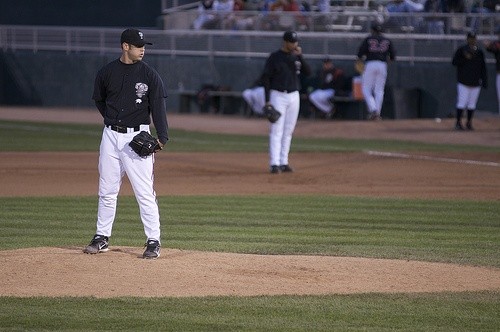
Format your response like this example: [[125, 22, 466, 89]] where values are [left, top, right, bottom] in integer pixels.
[[82, 234, 108, 254], [143, 237, 161, 260]]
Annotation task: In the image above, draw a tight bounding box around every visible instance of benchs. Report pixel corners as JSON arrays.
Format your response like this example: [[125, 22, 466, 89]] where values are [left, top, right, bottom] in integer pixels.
[[165, 89, 364, 120]]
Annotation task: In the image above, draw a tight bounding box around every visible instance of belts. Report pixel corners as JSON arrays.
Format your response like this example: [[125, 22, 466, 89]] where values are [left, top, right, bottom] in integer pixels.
[[277, 89, 297, 93], [106, 124, 139, 133]]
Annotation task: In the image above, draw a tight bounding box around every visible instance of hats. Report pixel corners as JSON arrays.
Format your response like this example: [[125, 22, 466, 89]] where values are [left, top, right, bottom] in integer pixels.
[[467, 32, 475, 39], [284, 31, 301, 41], [370, 21, 382, 31], [121, 29, 152, 48]]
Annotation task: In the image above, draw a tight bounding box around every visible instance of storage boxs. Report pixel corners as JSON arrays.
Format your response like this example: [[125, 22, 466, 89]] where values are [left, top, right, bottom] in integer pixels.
[[353, 77, 365, 98]]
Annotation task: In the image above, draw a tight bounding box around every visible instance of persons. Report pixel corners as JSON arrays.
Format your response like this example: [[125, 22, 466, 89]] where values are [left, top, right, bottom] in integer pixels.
[[192, 0, 500, 130], [83, 29, 168, 260], [264, 30, 313, 174]]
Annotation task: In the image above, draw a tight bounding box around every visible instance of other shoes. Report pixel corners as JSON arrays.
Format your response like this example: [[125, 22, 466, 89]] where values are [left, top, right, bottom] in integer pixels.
[[466, 120, 474, 130], [456, 123, 464, 131], [271, 166, 278, 173], [280, 165, 293, 173], [366, 112, 382, 121]]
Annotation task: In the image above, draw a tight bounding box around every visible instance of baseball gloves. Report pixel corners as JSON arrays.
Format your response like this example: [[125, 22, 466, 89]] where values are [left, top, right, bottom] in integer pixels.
[[129, 131, 161, 157], [262, 105, 281, 123]]
